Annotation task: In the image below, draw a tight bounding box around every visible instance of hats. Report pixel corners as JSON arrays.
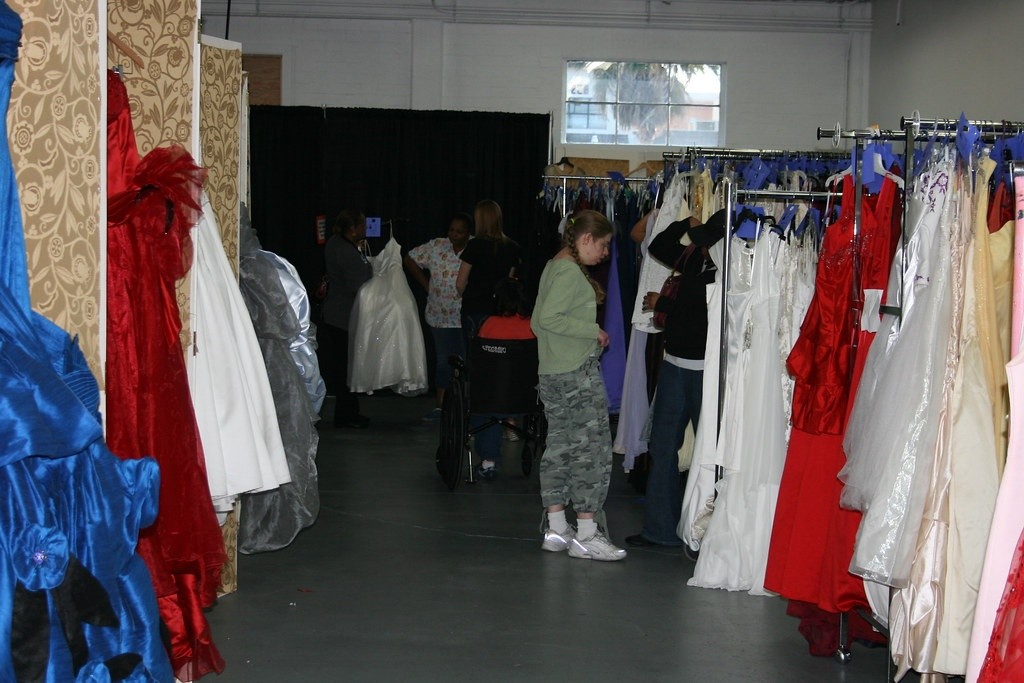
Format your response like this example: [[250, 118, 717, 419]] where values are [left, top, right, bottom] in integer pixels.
[[687, 208, 736, 247]]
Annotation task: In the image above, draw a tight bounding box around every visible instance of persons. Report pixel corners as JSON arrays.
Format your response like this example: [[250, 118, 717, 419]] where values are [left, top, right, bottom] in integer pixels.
[[625, 208, 737, 561], [313, 199, 535, 485], [530, 212, 628, 562]]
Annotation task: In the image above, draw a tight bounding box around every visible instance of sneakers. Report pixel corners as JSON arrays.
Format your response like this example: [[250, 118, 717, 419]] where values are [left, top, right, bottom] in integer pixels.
[[475, 462, 497, 481], [502, 429, 520, 442], [541, 524, 578, 552], [422, 406, 443, 422], [568, 529, 627, 562]]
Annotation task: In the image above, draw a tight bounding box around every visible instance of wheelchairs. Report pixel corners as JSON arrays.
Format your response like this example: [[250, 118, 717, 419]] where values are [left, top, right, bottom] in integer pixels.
[[436, 318, 548, 492]]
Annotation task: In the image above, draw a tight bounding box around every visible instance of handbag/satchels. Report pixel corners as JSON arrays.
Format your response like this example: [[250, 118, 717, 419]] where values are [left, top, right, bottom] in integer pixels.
[[653, 242, 696, 330]]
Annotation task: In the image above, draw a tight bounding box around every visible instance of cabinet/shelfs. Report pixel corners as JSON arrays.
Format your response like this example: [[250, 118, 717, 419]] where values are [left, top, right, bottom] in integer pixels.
[[0, 0, 241, 599]]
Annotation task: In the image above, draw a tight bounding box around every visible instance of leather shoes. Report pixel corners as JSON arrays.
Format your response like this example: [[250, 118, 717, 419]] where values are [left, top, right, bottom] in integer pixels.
[[624, 534, 682, 546]]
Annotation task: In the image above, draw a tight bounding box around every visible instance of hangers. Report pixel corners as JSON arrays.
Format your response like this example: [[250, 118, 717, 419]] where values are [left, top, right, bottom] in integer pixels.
[[349, 211, 396, 247], [536, 116, 1024, 238]]
